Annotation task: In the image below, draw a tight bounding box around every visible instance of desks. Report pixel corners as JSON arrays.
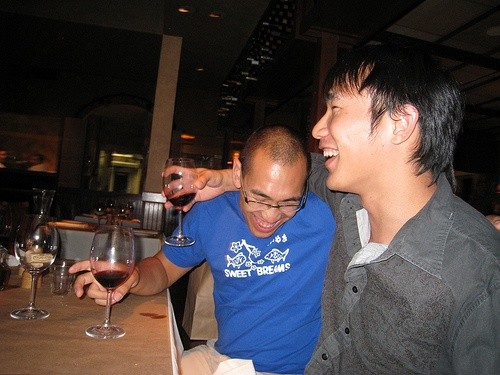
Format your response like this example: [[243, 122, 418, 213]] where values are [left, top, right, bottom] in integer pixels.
[[0, 263, 184, 375], [46, 221, 168, 261]]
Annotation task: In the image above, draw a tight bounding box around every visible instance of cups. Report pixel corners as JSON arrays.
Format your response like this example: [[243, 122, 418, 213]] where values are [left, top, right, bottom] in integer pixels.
[[32, 188, 56, 214], [50, 260, 77, 296]]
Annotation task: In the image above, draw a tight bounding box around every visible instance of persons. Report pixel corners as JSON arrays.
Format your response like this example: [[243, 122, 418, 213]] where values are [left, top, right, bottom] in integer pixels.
[[160, 47, 500, 375], [69, 125, 500, 375], [0, 150, 47, 171]]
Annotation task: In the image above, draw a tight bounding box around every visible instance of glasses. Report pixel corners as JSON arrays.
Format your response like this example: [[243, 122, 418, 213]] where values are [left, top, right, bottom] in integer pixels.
[[239, 170, 309, 213]]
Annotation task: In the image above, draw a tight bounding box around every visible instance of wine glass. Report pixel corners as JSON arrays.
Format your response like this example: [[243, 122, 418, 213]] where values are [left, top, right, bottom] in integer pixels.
[[84, 223, 135, 340], [163, 158, 199, 247], [0, 204, 13, 247], [95, 198, 134, 226], [10, 213, 59, 321]]
